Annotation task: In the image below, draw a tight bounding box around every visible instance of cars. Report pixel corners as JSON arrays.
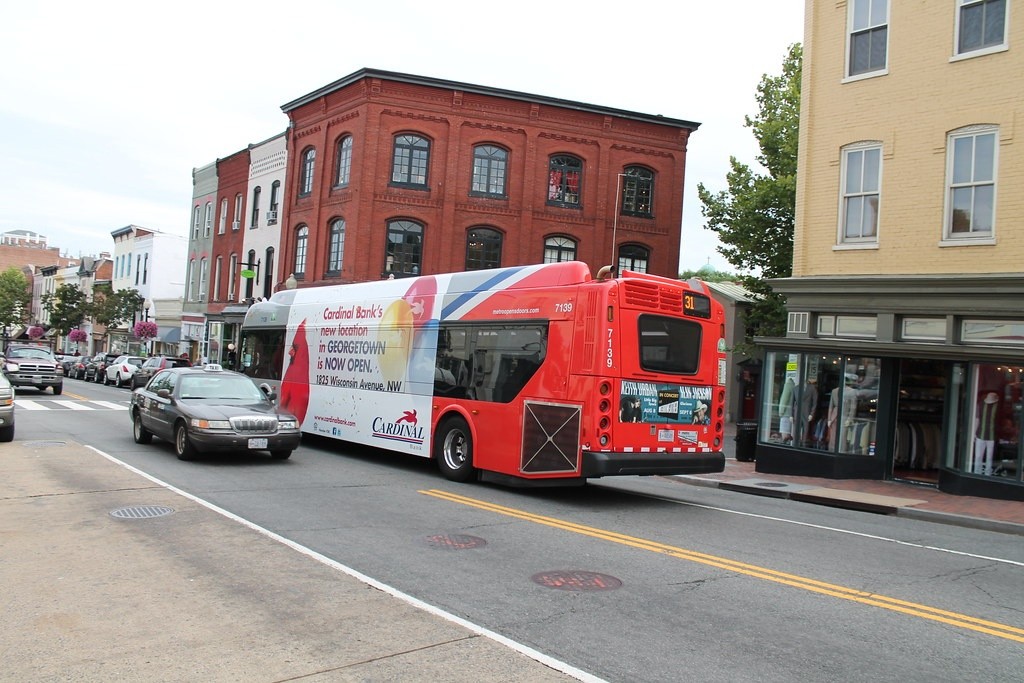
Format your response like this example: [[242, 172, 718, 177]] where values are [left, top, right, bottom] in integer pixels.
[[854, 377, 879, 414], [56, 355, 76, 374], [0, 363, 16, 442], [129, 363, 302, 462], [68, 356, 95, 378]]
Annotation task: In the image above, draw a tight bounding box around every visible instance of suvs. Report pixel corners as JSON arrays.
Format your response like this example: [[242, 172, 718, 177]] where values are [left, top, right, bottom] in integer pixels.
[[84, 352, 124, 383], [130, 357, 193, 392], [103, 354, 150, 388]]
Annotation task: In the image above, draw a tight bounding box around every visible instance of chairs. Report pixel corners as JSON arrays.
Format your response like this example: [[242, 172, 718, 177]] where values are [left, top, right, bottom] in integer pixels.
[[179, 387, 200, 398]]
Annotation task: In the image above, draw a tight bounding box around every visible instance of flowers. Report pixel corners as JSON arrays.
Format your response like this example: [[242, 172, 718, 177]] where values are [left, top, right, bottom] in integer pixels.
[[70, 330, 86, 342], [134, 321, 157, 338]]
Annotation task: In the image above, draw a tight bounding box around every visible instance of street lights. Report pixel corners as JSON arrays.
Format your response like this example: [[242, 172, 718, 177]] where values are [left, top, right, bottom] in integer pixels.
[[610, 172, 630, 279], [144, 301, 151, 355]]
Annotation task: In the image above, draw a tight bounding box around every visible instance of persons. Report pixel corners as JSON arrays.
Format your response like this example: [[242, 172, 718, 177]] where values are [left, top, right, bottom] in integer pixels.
[[789, 379, 818, 447], [778, 378, 795, 448], [74, 350, 82, 357], [619, 399, 641, 423], [693, 403, 710, 425], [972, 392, 1003, 477], [828, 378, 857, 453]]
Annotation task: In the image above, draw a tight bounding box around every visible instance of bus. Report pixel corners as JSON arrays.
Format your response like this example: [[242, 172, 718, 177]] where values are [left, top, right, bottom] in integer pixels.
[[236, 261, 725, 487]]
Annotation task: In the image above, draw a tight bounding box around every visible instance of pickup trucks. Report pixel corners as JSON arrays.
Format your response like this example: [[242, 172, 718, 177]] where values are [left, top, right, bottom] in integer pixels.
[[0, 344, 64, 395]]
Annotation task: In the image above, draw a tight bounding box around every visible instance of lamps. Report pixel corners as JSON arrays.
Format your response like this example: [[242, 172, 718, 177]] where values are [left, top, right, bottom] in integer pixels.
[[237, 258, 261, 285]]
